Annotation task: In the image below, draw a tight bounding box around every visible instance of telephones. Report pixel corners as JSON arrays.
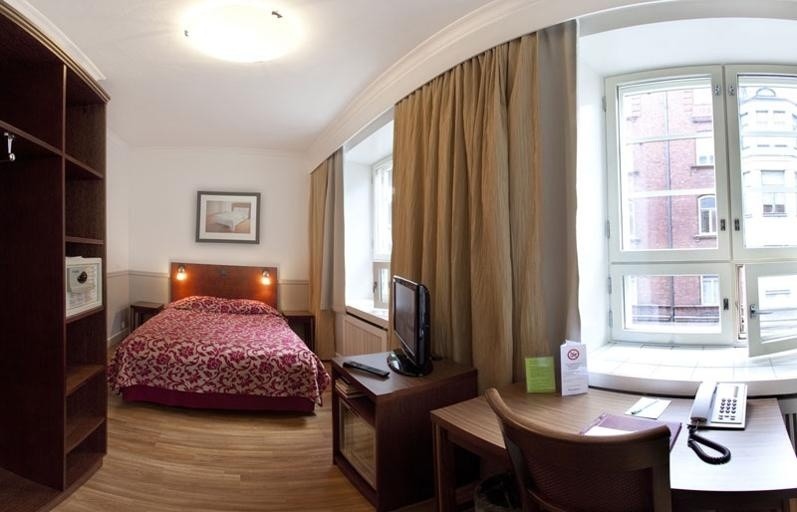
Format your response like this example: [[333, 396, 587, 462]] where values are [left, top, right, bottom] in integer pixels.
[[687, 380, 748, 431]]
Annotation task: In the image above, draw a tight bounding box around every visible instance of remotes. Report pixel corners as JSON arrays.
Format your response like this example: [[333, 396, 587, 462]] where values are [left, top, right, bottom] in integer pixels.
[[343, 360, 390, 377]]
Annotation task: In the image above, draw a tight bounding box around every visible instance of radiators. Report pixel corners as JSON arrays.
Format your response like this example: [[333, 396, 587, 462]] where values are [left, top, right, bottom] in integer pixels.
[[335, 316, 386, 360]]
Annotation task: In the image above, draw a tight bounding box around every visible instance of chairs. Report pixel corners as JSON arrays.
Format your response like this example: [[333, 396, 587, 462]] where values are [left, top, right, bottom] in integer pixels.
[[484, 387, 671, 510]]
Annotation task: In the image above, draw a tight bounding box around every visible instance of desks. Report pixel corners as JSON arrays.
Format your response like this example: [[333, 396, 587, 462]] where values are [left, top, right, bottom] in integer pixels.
[[429, 379, 797, 512]]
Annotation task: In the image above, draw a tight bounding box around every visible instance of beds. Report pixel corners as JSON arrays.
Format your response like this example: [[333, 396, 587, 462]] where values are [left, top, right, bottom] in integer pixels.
[[208, 202, 251, 232], [123, 262, 314, 413]]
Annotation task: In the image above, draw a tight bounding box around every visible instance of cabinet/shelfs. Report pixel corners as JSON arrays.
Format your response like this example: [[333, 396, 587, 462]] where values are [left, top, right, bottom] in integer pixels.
[[332, 354, 479, 506], [63, 52, 107, 487], [0, 2, 62, 512]]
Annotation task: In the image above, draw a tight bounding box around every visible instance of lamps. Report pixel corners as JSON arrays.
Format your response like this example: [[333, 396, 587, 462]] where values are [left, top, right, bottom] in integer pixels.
[[261, 268, 271, 285], [175, 264, 187, 282]]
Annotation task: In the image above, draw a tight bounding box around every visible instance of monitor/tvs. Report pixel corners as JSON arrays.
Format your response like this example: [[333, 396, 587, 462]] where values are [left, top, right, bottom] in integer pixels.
[[387, 275, 433, 377]]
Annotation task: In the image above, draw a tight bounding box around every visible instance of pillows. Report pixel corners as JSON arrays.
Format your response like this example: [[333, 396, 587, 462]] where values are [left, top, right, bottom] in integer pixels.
[[172, 292, 220, 313], [226, 295, 271, 316]]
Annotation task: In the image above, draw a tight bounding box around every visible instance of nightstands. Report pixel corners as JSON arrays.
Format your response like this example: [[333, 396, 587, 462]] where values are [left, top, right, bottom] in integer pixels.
[[280, 310, 315, 356], [130, 301, 164, 337]]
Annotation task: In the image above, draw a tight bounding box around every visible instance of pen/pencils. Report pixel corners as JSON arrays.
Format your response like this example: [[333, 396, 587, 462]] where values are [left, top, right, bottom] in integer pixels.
[[631, 399, 658, 415]]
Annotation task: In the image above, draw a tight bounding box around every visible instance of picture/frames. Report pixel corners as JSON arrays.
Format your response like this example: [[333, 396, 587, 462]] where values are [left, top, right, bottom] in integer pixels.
[[196, 190, 260, 243]]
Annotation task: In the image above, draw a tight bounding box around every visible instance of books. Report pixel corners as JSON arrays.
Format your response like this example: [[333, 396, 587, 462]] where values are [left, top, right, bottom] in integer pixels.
[[576, 411, 684, 454]]
